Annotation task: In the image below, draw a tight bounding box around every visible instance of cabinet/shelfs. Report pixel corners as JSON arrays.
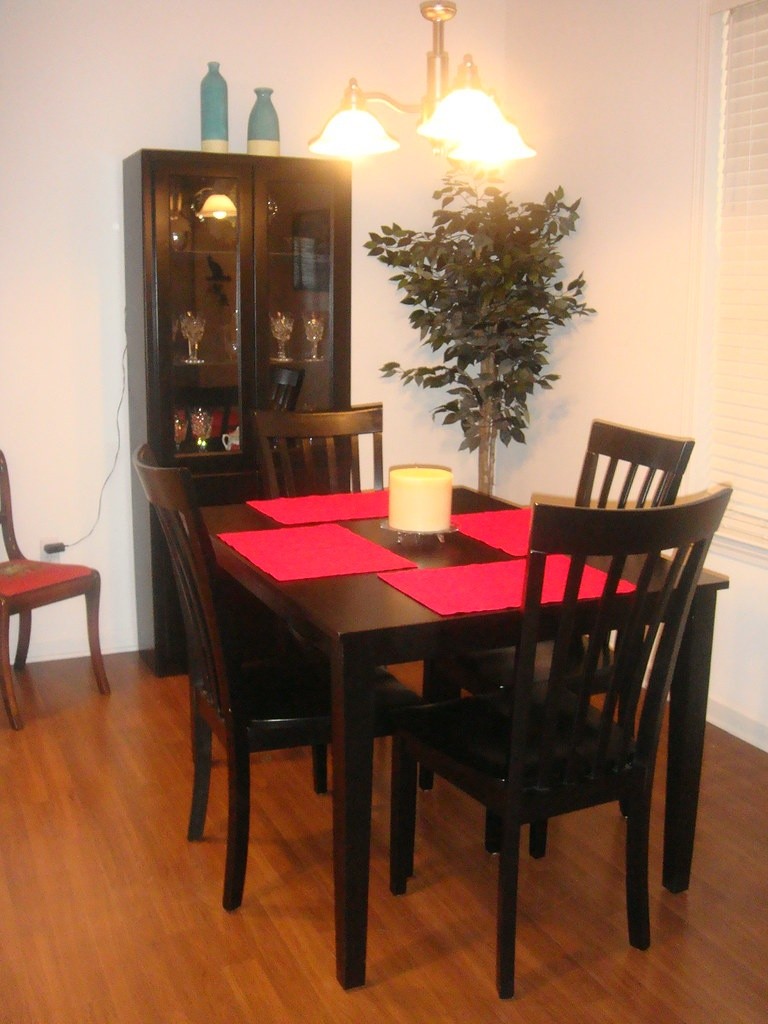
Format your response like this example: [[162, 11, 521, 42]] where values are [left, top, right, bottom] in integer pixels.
[[122, 148, 352, 676]]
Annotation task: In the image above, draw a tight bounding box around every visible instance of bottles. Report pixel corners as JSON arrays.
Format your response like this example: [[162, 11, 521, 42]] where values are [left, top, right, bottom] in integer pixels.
[[271, 369, 303, 412]]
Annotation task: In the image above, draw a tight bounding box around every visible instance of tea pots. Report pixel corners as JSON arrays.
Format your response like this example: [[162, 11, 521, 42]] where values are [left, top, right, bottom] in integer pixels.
[[222, 426, 240, 451]]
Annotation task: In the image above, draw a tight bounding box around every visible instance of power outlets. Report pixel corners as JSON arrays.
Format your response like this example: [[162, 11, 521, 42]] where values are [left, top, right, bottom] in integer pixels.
[[40, 541, 60, 564]]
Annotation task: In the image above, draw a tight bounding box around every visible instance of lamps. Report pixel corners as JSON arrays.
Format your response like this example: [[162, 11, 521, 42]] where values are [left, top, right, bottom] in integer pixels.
[[380, 463, 459, 545], [308, 3, 537, 168], [195, 193, 237, 219]]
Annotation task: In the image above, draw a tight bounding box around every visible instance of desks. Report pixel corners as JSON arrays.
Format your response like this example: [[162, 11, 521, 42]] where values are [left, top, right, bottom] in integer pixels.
[[179, 487, 729, 990]]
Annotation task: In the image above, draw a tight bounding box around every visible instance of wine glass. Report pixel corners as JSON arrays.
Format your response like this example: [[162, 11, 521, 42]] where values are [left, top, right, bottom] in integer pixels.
[[304, 311, 327, 362], [180, 311, 207, 364], [191, 408, 212, 453], [269, 310, 294, 362], [174, 409, 188, 452]]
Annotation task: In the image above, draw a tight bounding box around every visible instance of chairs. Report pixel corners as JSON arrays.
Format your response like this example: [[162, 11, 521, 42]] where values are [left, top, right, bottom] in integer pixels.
[[459, 419, 693, 699], [384, 482, 732, 1001], [250, 403, 384, 498], [0, 448, 112, 731], [132, 442, 425, 912]]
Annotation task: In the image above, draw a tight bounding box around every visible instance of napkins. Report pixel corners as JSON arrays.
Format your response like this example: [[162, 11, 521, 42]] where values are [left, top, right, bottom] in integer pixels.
[[247, 490, 389, 525], [377, 554, 638, 617], [217, 521, 418, 583], [450, 506, 533, 557]]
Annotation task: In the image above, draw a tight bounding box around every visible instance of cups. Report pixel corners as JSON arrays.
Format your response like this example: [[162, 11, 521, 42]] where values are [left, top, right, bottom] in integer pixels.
[[170, 232, 187, 250]]
